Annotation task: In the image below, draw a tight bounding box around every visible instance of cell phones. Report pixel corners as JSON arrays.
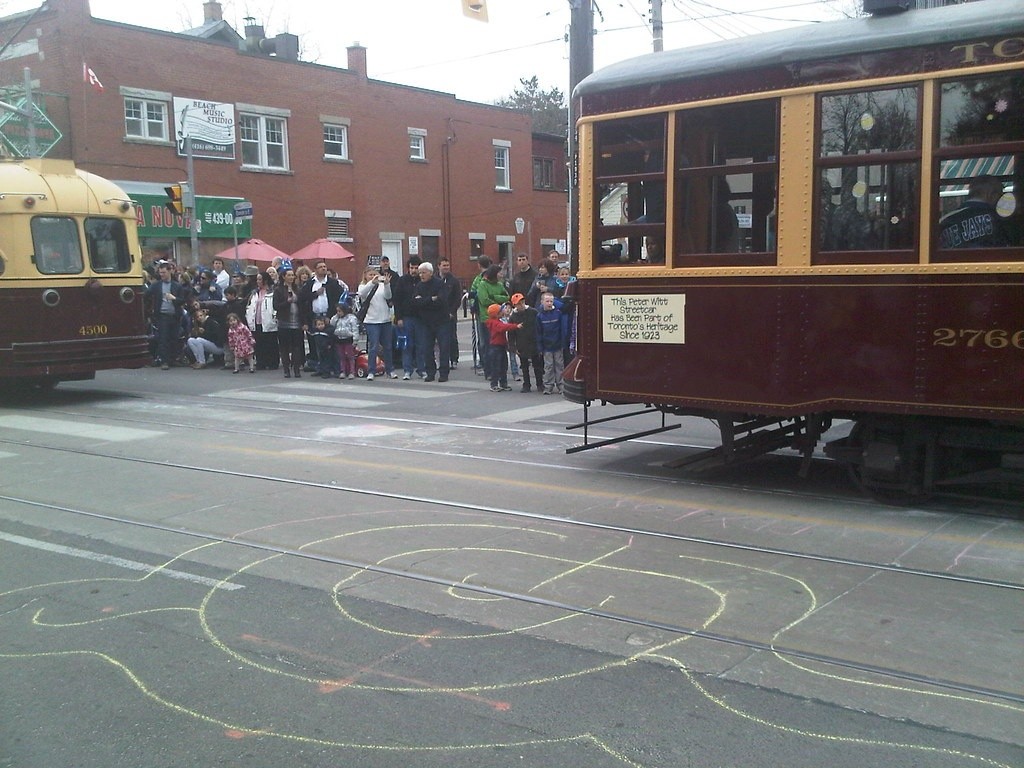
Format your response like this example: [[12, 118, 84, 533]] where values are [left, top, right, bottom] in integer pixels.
[[378, 276, 385, 281]]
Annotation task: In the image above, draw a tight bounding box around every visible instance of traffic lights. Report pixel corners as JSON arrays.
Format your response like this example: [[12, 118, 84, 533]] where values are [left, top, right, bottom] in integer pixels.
[[164, 186, 181, 215]]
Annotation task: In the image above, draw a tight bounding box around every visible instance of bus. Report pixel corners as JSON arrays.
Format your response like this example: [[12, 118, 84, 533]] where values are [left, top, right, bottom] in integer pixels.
[[560, 0, 1024, 510], [1, 158, 149, 392]]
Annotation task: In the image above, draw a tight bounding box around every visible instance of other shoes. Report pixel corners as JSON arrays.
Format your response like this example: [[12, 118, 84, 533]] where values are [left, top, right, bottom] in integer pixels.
[[512, 373, 521, 381], [500, 385, 512, 391], [520, 383, 531, 393], [474, 364, 482, 369], [537, 385, 546, 392], [477, 370, 485, 375], [486, 371, 492, 380], [154, 346, 461, 384], [557, 384, 564, 394], [490, 385, 502, 392], [542, 388, 554, 395]]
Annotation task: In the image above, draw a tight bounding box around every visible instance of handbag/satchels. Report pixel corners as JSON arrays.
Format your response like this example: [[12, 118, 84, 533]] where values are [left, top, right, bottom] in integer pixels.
[[356, 300, 370, 323]]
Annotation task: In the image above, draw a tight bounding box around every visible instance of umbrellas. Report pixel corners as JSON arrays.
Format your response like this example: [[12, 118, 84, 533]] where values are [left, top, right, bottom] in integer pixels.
[[290, 237, 353, 264], [214, 238, 292, 267]]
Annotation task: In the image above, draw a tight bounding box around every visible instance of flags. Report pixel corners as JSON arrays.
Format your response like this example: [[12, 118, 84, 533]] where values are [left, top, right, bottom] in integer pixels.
[[84, 64, 105, 94]]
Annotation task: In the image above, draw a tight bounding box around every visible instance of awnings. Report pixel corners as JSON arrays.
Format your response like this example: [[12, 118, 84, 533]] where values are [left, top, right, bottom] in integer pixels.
[[876, 154, 1015, 203]]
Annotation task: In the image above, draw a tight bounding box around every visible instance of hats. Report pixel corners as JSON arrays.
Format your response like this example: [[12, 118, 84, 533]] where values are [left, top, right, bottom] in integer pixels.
[[501, 301, 512, 308], [231, 271, 242, 277], [511, 293, 525, 305], [380, 256, 389, 261], [243, 265, 260, 275], [488, 303, 505, 318]]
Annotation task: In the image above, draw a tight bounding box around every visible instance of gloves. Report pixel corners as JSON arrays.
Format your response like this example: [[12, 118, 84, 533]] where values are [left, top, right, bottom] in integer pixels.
[[351, 340, 358, 349]]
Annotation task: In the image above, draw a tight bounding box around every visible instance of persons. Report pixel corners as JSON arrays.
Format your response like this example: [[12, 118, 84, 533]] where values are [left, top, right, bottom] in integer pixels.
[[819, 179, 844, 251], [937, 175, 1009, 248], [462, 250, 577, 395], [144, 253, 462, 382]]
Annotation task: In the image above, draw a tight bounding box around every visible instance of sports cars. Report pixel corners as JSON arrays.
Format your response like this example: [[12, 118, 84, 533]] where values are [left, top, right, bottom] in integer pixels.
[[353, 344, 385, 378]]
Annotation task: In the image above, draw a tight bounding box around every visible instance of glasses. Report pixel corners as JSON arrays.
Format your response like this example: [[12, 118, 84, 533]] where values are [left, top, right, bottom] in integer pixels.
[[199, 275, 206, 280]]
